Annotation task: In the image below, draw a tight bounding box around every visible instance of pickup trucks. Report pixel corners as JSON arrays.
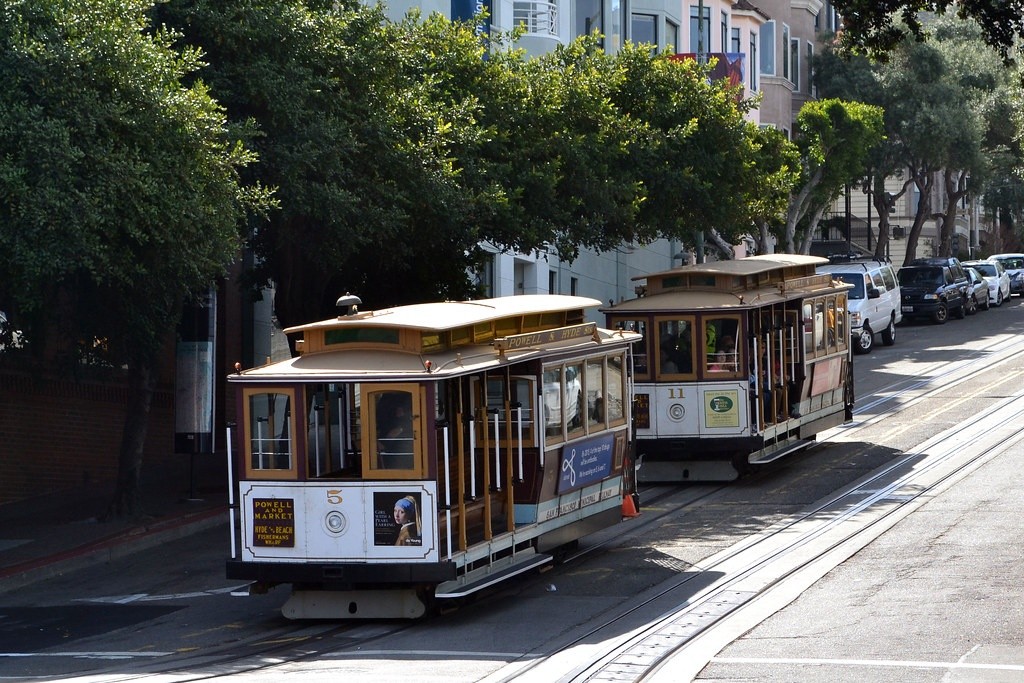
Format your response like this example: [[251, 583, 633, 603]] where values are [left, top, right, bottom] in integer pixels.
[[896, 257, 970, 324]]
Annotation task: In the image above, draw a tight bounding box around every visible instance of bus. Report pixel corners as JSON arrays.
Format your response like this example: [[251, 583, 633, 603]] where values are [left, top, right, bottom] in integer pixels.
[[221, 291, 644, 622], [598, 253, 855, 486]]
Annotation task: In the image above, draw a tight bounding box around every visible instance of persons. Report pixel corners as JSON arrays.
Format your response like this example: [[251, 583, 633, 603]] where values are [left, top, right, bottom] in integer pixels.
[[376, 392, 412, 451], [678, 320, 716, 362], [394, 496, 422, 546], [709, 333, 791, 422], [635, 334, 679, 374]]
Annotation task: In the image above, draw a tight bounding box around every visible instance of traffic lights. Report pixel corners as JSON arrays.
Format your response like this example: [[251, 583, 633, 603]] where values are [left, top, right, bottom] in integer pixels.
[[884, 192, 896, 213]]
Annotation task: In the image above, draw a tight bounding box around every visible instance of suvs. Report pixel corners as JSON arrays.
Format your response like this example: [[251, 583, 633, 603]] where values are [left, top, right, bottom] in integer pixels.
[[961, 258, 1011, 306]]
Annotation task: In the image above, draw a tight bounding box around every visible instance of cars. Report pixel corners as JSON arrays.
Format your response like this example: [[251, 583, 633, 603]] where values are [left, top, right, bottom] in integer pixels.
[[961, 266, 990, 314]]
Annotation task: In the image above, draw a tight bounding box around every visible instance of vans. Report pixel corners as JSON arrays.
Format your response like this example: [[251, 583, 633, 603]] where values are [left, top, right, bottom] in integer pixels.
[[815, 254, 904, 354], [986, 253, 1024, 297]]
